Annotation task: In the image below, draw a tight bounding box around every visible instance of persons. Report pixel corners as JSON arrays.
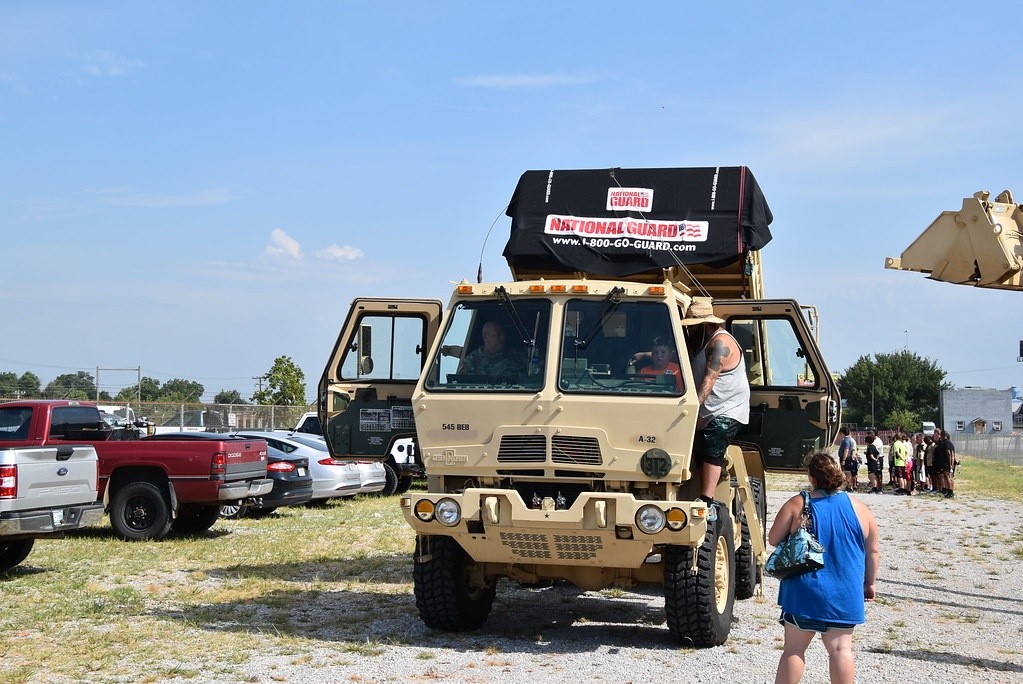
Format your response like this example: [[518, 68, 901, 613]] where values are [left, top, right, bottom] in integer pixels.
[[838, 427, 960, 499], [636, 336, 684, 391], [627, 297, 750, 521], [456, 321, 527, 376], [769, 454, 879, 684]]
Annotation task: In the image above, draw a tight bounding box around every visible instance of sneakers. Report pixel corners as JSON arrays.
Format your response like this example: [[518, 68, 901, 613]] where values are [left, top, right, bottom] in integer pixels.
[[694, 498, 718, 522]]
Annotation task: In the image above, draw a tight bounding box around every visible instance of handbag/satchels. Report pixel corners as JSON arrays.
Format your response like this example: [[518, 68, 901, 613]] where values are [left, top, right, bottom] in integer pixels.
[[766, 491, 826, 580]]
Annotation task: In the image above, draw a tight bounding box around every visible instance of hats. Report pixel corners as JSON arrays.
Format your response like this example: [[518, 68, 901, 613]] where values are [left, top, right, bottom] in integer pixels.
[[681, 296, 726, 326]]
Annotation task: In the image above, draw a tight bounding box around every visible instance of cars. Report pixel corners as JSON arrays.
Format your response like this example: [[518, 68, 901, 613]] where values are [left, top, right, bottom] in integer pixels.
[[297, 431, 386, 498], [219, 430, 361, 507], [140, 430, 314, 518]]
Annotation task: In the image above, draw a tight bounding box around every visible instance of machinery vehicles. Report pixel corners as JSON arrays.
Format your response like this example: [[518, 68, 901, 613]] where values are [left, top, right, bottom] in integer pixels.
[[316, 167, 849, 648]]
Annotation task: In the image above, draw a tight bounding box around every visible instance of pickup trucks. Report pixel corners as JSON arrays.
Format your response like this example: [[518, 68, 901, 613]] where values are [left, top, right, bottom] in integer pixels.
[[0, 444, 104, 567], [1, 396, 275, 540]]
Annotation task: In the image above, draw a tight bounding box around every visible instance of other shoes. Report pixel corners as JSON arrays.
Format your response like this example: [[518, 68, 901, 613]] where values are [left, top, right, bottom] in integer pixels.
[[895, 488, 905, 492], [868, 488, 878, 494], [945, 491, 953, 498]]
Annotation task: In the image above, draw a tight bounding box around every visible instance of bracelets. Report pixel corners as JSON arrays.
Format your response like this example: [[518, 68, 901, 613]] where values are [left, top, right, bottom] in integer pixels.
[[842, 459, 845, 461]]
